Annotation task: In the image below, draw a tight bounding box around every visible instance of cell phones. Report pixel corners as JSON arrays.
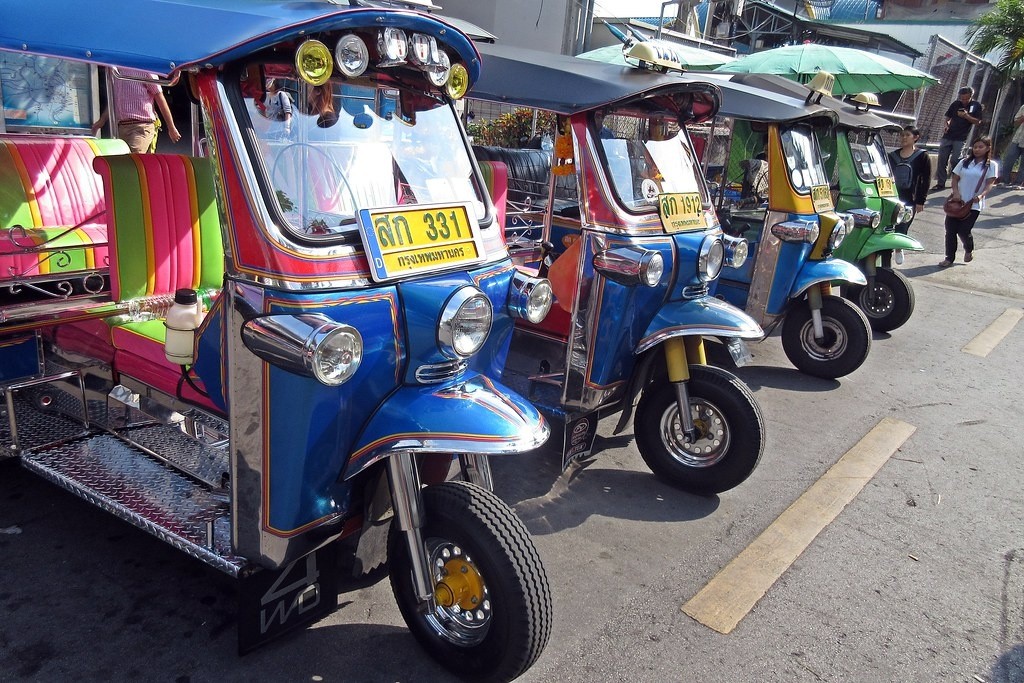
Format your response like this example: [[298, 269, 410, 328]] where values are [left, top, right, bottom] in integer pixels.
[[959, 108, 964, 112]]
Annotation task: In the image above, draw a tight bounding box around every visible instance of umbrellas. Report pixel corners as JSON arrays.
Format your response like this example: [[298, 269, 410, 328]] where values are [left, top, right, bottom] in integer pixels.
[[561, 35, 943, 96]]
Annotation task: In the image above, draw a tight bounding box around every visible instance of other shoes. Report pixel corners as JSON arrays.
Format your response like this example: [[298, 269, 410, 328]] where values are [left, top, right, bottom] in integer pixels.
[[933, 185, 945, 190], [1012, 182, 1024, 190], [997, 182, 1012, 188]]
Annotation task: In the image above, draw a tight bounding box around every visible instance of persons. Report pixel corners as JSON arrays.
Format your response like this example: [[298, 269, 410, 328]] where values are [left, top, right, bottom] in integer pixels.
[[886, 87, 999, 266], [254, 74, 340, 142], [997, 105, 1024, 190], [90, 65, 182, 158], [591, 109, 615, 139]]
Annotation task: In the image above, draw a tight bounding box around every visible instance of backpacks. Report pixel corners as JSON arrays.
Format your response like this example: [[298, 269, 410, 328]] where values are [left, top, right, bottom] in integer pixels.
[[889, 149, 927, 190]]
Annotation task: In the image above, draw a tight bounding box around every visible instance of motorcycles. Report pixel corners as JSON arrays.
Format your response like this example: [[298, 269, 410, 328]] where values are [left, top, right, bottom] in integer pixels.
[[1, 0, 555, 683], [732, 66, 926, 332], [467, 38, 767, 498], [664, 68, 874, 380]]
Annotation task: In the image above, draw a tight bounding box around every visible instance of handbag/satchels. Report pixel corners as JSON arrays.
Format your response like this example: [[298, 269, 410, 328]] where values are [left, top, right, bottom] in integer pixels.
[[943, 192, 974, 218]]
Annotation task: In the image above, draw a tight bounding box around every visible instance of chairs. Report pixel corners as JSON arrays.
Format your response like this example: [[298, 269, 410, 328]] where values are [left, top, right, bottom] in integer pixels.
[[55, 304, 133, 367], [475, 160, 509, 247], [92, 153, 226, 419]]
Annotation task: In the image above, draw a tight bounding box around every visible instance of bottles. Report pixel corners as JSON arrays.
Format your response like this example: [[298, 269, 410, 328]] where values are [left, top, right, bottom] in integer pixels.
[[164, 288, 204, 365]]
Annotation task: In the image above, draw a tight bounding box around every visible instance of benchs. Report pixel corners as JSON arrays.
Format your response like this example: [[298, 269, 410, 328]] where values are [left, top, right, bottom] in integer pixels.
[[472, 145, 580, 217], [0, 137, 132, 281]]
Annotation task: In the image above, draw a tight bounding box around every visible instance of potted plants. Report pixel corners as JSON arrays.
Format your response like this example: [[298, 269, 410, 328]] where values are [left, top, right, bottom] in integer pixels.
[[999, 150, 1021, 183]]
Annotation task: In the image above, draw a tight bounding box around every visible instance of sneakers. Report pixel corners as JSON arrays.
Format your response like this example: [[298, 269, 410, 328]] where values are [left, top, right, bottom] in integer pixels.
[[895, 249, 903, 264], [964, 251, 974, 262], [939, 260, 954, 268]]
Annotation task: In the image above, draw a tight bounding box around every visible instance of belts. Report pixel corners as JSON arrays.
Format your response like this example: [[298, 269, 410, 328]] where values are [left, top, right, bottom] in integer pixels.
[[118, 120, 142, 125]]
